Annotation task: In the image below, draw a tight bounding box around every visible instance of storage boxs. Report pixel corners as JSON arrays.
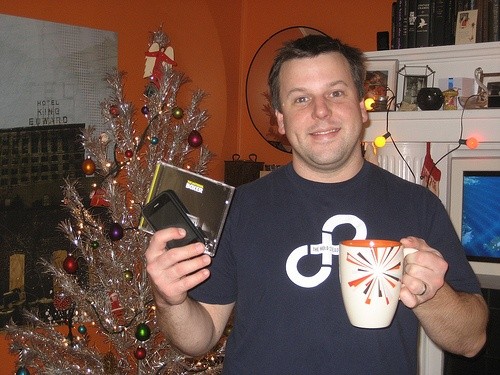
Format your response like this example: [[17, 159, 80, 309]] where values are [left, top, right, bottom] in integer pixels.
[[224, 154, 265, 187]]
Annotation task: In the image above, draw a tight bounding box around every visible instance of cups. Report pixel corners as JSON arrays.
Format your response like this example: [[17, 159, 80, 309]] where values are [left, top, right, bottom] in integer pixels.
[[338, 239, 419, 328]]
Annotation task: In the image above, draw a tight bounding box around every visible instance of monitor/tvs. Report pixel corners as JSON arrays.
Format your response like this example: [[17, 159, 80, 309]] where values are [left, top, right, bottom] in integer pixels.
[[447, 157, 500, 290]]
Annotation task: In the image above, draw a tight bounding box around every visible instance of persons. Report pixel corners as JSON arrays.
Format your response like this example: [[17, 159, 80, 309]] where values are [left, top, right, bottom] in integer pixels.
[[144, 34, 490, 374]]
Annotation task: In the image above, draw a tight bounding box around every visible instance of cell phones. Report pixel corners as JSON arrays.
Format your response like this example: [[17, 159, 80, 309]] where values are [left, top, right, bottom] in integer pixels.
[[142, 190, 205, 263]]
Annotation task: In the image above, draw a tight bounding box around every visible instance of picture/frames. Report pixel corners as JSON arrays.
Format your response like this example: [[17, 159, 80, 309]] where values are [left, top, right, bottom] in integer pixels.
[[361, 59, 399, 112], [396, 64, 435, 111]]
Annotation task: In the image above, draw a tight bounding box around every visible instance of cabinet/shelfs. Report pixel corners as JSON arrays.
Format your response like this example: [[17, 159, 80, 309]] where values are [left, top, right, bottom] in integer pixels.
[[359, 41, 500, 375]]
